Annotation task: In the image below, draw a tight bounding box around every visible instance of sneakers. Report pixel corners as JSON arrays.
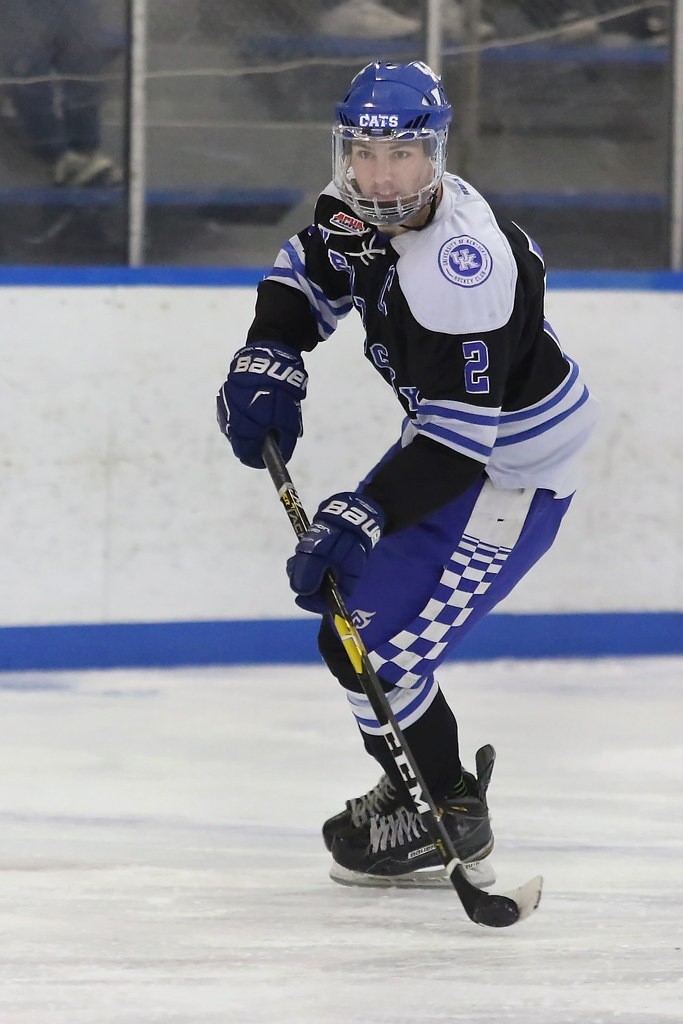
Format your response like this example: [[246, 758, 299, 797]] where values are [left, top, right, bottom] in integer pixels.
[[322, 771, 403, 852], [329, 744, 498, 889]]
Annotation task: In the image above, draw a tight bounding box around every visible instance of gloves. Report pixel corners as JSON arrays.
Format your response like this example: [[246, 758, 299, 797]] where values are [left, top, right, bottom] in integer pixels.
[[285, 494, 385, 615], [215, 343, 310, 470]]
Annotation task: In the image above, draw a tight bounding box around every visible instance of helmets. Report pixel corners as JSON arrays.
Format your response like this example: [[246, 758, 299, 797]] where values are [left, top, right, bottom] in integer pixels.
[[330, 60, 451, 226]]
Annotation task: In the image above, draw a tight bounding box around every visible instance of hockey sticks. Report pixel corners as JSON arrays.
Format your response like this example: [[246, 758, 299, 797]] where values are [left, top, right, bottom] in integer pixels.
[[262, 437, 546, 929]]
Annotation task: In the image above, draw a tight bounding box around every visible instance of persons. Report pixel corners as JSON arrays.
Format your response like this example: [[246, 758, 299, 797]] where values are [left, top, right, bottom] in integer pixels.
[[295, 0, 672, 47], [212, 58, 595, 893], [0, 0, 111, 244]]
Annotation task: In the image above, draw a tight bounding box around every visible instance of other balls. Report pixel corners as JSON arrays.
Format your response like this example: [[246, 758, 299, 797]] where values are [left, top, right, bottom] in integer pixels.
[[474, 894, 520, 928]]
